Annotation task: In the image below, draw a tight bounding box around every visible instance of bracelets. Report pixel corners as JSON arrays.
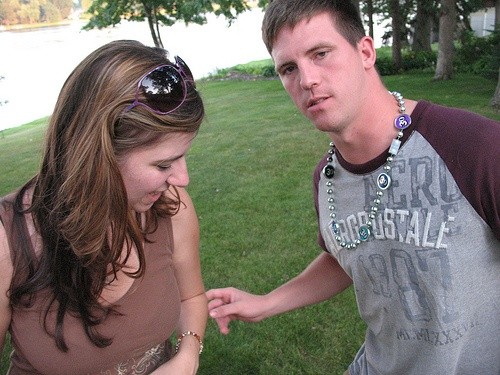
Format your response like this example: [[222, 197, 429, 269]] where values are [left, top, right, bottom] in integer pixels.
[[175, 330, 204, 356]]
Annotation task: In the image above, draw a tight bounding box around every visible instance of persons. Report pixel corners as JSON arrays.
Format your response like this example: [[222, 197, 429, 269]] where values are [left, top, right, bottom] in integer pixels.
[[1, 39, 211, 375], [204, 1, 500, 375]]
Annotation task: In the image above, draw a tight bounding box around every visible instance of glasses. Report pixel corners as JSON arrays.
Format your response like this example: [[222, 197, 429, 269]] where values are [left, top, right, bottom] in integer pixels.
[[121, 56, 194, 116]]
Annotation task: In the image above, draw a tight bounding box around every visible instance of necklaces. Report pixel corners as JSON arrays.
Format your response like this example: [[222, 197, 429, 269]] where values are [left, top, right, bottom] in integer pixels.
[[322, 89, 414, 249]]
